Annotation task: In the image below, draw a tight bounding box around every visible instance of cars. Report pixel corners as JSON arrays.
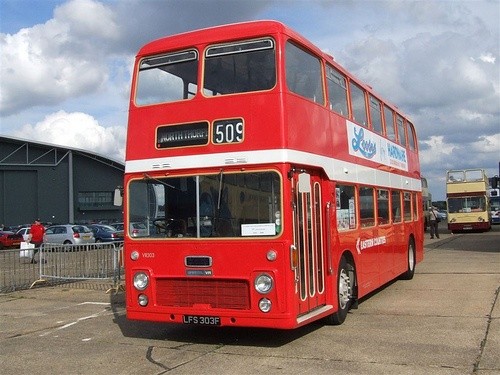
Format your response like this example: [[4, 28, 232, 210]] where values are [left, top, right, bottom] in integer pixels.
[[84, 223, 124, 250], [41, 224, 96, 253], [15, 227, 54, 243], [110, 222, 149, 240], [428, 207, 447, 220], [0, 230, 25, 250]]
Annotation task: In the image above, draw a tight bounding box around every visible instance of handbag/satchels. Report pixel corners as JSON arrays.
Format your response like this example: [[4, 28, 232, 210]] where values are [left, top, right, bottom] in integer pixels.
[[20, 242, 35, 257], [436, 217, 441, 223]]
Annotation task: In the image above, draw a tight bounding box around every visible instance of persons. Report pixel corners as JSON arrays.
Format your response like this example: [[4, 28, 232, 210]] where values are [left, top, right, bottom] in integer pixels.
[[28, 219, 47, 264], [428, 206, 440, 239]]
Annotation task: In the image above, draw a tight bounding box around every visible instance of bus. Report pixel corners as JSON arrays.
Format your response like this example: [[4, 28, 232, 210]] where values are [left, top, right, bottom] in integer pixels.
[[114, 20, 424, 332], [446, 168, 492, 233], [488, 175, 500, 225]]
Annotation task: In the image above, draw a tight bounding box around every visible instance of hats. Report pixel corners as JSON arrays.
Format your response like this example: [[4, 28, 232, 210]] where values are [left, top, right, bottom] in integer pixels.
[[35, 218, 41, 222]]
